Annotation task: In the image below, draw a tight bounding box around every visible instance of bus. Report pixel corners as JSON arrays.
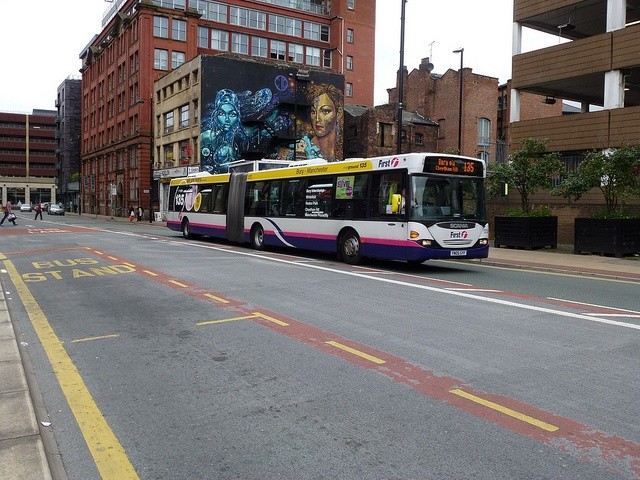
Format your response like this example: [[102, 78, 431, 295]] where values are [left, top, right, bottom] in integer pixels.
[[167, 152, 490, 266]]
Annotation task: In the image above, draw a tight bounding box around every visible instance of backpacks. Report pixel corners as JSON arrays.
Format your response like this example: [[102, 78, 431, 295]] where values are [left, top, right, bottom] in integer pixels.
[[34, 206, 39, 212]]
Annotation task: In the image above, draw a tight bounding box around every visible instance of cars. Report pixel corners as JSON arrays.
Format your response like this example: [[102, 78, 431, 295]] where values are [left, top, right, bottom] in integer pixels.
[[34, 202, 49, 212], [21, 204, 32, 212], [48, 203, 65, 215]]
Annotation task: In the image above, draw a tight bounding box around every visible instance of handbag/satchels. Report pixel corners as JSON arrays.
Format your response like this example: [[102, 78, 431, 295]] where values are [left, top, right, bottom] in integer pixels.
[[7, 213, 17, 221]]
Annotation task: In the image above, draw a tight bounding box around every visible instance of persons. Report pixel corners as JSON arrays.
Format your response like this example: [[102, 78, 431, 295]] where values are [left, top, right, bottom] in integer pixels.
[[201, 88, 249, 166], [306, 83, 344, 161], [398, 184, 420, 209], [0, 197, 18, 226], [34, 199, 43, 220], [127, 204, 144, 223], [259, 191, 269, 202], [56, 200, 78, 213]]
